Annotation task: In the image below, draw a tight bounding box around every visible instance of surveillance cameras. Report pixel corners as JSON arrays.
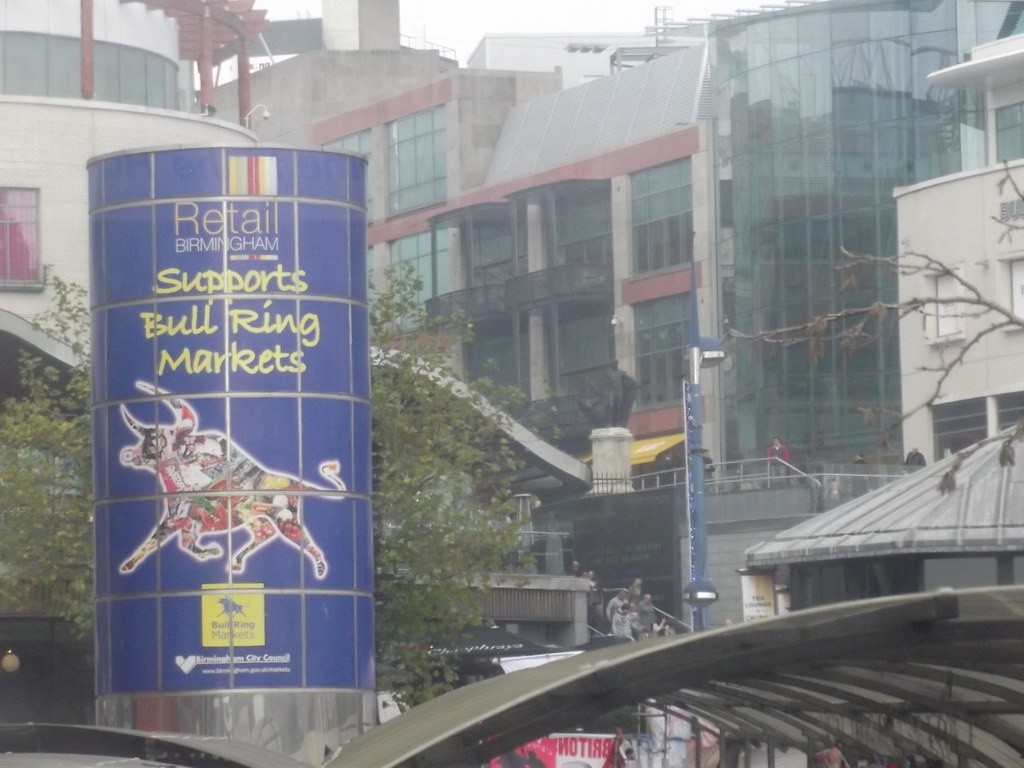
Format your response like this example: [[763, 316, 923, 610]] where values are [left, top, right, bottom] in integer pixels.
[[263, 111, 270, 119]]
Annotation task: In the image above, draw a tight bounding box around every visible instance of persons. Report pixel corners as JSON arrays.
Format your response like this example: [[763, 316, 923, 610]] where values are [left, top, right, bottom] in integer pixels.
[[580, 568, 673, 644], [702, 449, 718, 495], [848, 453, 869, 501], [766, 436, 790, 487], [903, 444, 926, 477]]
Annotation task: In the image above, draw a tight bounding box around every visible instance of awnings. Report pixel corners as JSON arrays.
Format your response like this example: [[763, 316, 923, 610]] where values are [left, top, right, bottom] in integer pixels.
[[578, 429, 685, 464]]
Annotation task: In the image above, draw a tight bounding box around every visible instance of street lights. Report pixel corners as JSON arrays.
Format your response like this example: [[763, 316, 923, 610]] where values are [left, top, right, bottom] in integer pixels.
[[682, 346, 726, 633]]
